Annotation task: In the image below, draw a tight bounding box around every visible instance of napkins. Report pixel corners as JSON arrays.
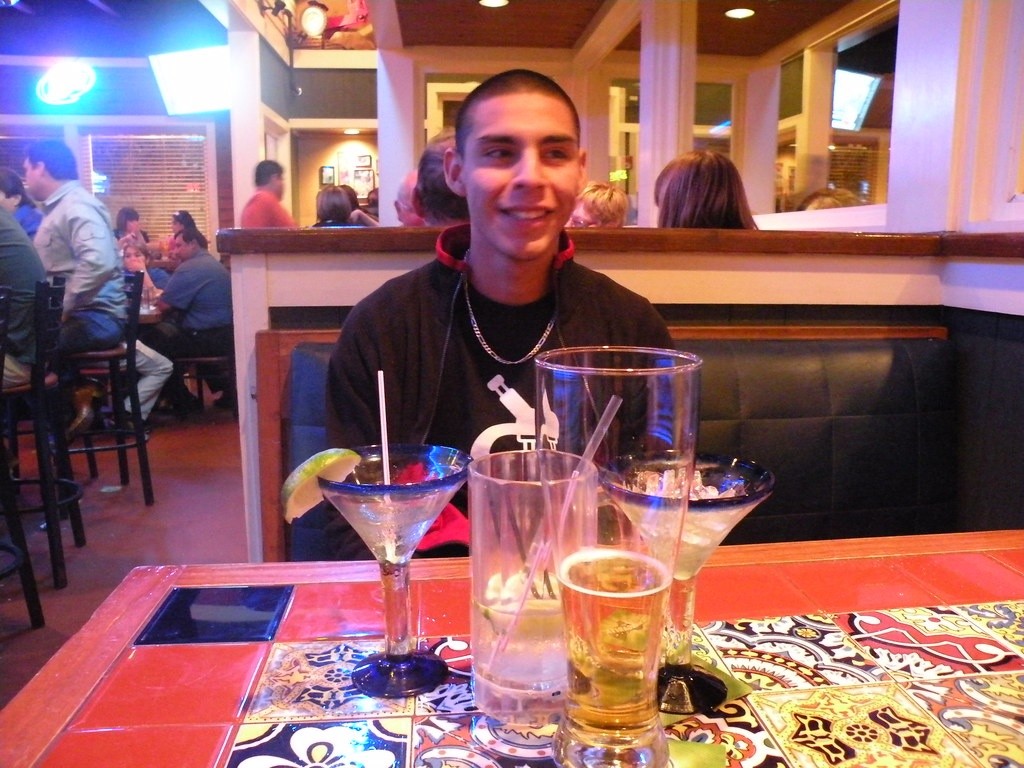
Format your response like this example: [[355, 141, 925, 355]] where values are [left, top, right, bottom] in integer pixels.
[[392, 458, 471, 550]]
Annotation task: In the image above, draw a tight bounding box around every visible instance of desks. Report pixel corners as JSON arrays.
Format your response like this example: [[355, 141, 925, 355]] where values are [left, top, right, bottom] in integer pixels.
[[0, 529, 1024, 768]]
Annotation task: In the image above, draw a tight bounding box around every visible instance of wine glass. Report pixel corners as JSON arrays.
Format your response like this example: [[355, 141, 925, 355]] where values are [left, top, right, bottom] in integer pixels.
[[600, 446, 778, 717], [316, 441, 476, 700]]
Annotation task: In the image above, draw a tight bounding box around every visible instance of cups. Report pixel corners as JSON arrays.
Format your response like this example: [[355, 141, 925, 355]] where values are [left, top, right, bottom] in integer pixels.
[[466, 449, 600, 729], [534, 346, 704, 767]]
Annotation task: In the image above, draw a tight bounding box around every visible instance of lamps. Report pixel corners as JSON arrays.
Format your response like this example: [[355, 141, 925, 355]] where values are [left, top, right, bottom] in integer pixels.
[[293, 5, 328, 50]]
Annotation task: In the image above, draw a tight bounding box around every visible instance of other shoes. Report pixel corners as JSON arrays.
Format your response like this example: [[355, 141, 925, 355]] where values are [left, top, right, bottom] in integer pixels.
[[65, 388, 94, 442], [104, 415, 152, 434]]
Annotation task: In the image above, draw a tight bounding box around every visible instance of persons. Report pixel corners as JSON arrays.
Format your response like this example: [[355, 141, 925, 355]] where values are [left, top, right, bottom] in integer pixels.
[[240, 160, 295, 228], [564, 181, 628, 228], [0, 137, 238, 445], [311, 185, 380, 227], [411, 126, 471, 227], [394, 168, 426, 228], [796, 187, 864, 213], [325, 68, 677, 563], [652, 149, 759, 230]]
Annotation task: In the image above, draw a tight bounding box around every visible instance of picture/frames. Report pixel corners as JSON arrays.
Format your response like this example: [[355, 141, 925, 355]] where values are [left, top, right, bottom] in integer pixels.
[[321, 166, 335, 185], [356, 155, 371, 167], [353, 169, 375, 200]]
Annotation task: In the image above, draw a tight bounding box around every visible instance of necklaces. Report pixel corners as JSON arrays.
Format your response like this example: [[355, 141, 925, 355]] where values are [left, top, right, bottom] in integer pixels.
[[462, 248, 560, 365]]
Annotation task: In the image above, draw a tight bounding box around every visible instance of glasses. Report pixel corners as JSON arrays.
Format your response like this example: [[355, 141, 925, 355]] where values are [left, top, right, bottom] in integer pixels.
[[571, 218, 597, 227]]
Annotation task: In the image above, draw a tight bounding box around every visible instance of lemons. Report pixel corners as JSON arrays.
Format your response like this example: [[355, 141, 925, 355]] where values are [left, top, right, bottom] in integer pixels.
[[481, 602, 565, 639], [280, 448, 362, 522]]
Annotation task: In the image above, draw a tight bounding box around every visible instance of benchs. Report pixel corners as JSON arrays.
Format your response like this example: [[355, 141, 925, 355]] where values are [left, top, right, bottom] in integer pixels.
[[256, 326, 965, 562]]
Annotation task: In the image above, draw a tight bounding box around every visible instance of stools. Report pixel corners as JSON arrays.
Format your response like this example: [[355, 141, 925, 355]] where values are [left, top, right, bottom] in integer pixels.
[[58, 270, 154, 516], [1, 272, 88, 629]]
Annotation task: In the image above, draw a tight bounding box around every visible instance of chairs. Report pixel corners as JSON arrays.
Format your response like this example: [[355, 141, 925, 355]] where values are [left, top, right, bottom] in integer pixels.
[[173, 350, 236, 410], [76, 364, 125, 378]]
